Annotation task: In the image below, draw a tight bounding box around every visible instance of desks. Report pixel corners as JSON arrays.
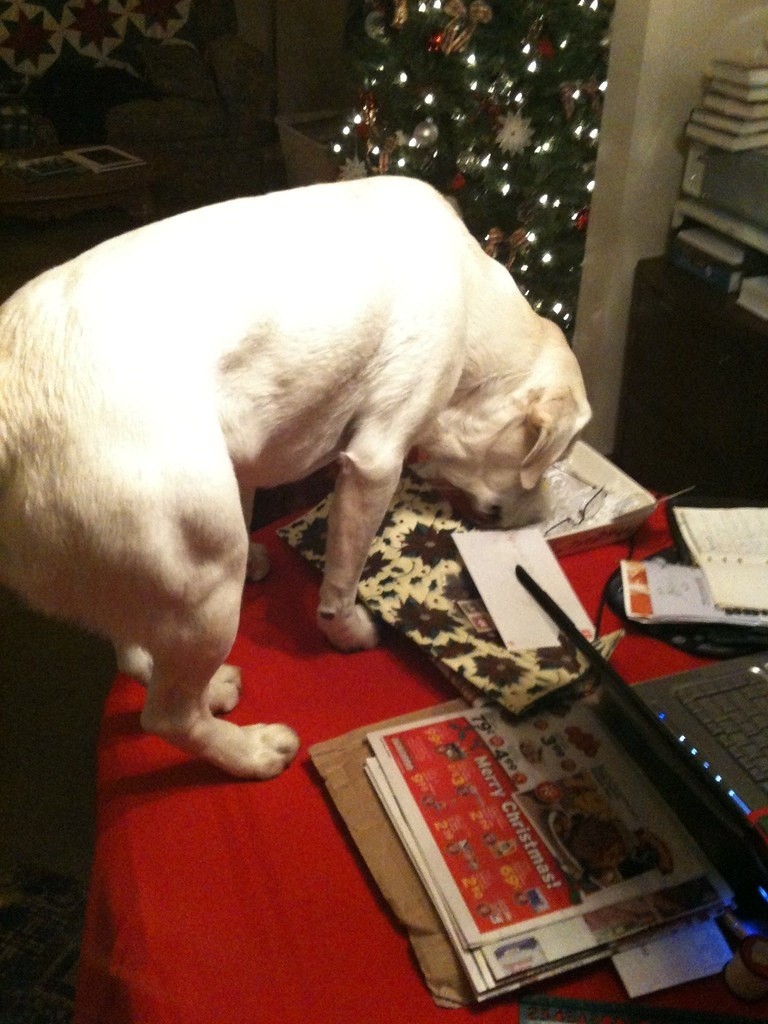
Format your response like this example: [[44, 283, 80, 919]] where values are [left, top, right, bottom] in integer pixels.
[[76, 482, 768, 1024]]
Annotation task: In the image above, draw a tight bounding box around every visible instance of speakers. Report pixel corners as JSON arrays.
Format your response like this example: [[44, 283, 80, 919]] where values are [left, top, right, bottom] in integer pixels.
[[609, 258, 767, 508]]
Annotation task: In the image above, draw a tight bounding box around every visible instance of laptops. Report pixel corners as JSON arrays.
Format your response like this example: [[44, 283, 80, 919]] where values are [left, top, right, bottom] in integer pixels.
[[516, 565, 768, 931]]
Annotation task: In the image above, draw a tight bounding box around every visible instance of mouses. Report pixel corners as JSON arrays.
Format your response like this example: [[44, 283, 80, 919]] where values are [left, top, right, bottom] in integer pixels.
[[604, 546, 680, 621]]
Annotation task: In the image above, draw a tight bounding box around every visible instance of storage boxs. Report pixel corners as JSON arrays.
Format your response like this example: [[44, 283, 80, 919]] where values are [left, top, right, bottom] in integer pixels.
[[276, 105, 361, 186]]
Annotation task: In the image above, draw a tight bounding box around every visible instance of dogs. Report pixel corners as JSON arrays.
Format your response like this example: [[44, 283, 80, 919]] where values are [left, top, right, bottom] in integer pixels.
[[0, 174, 593, 782]]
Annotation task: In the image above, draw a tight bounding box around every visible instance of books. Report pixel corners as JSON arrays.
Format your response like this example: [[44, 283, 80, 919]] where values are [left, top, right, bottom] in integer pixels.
[[682, 48, 767, 154], [674, 507, 768, 617]]
